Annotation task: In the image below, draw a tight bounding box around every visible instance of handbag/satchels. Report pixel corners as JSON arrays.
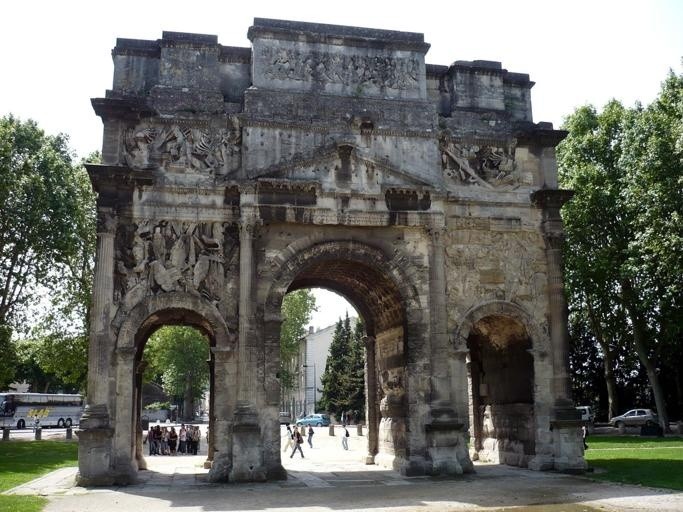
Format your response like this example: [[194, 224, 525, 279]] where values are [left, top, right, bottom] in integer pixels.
[[298, 435, 303, 443], [346, 430, 349, 436]]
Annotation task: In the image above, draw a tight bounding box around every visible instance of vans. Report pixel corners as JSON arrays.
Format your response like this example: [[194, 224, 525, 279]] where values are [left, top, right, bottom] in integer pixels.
[[575, 406, 594, 422], [279, 411, 290, 425], [295, 413, 329, 427]]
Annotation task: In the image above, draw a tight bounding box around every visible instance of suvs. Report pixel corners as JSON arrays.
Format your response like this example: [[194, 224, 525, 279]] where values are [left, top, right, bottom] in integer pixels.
[[607, 408, 657, 428]]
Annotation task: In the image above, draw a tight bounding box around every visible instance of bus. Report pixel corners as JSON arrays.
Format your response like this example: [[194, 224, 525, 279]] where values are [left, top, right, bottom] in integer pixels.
[[0, 392, 85, 428]]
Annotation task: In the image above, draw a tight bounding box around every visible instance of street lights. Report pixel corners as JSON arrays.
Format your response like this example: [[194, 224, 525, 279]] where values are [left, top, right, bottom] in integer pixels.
[[301, 362, 316, 412]]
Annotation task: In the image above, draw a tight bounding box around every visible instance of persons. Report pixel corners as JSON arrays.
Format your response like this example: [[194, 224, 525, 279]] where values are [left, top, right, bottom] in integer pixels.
[[306, 423, 314, 448], [340, 424, 348, 450], [282, 422, 293, 452], [142, 424, 201, 455], [32, 414, 39, 433], [290, 427, 304, 459], [580, 421, 588, 450]]
